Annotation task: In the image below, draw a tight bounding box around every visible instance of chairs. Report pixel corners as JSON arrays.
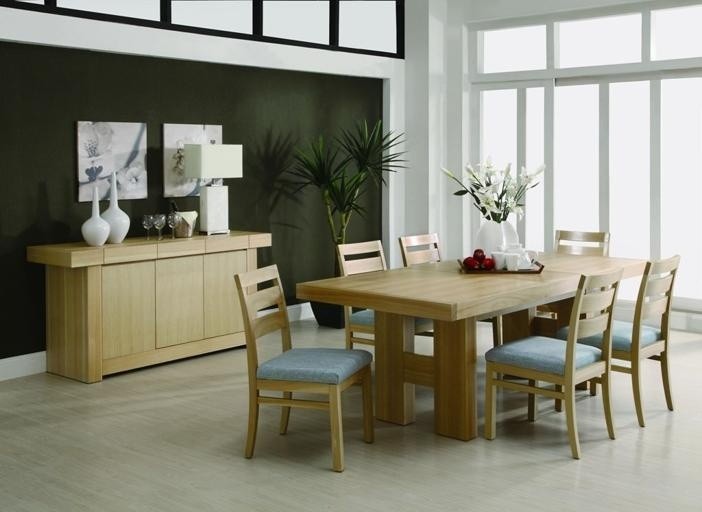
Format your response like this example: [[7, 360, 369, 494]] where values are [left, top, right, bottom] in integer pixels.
[[537, 229, 613, 326], [398, 232, 444, 269], [552, 255, 682, 429], [335, 237, 389, 360], [483, 266, 623, 461], [234, 262, 378, 474]]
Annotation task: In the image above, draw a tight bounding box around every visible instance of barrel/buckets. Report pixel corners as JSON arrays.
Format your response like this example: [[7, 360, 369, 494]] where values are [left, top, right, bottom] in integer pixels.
[[174, 211, 198, 238]]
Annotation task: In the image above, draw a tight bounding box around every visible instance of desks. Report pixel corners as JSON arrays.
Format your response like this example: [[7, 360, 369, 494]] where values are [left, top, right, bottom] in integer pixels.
[[297, 256, 652, 441], [26, 224, 275, 384]]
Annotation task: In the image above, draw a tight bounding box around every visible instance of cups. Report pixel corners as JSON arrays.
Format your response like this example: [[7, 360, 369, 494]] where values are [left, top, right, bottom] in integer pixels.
[[492, 251, 506, 271], [502, 253, 524, 272]]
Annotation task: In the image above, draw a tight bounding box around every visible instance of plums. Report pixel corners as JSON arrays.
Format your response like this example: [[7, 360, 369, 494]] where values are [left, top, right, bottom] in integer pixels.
[[463, 248, 494, 270]]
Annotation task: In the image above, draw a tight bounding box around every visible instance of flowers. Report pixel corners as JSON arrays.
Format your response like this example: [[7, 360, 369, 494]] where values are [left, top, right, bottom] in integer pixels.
[[440, 158, 546, 222]]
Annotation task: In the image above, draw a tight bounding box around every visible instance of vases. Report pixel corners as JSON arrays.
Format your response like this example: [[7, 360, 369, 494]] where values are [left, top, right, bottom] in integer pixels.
[[473, 221, 519, 258], [101, 172, 131, 243], [79, 185, 110, 247]]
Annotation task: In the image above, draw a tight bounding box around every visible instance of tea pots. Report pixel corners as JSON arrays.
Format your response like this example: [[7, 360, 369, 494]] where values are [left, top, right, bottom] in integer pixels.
[[497, 243, 538, 270]]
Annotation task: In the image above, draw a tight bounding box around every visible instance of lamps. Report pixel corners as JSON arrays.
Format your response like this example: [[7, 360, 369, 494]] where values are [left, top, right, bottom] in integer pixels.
[[182, 144, 244, 236]]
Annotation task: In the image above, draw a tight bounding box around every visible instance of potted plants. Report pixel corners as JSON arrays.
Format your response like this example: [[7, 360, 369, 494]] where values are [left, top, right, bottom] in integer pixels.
[[282, 113, 413, 331]]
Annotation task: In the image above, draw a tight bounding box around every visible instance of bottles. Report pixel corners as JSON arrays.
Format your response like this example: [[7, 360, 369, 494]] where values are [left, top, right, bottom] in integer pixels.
[[170, 200, 179, 212]]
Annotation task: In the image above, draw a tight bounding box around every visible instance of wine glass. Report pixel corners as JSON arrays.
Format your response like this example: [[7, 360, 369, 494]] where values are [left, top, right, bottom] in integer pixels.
[[140, 214, 153, 241], [152, 214, 167, 241], [167, 214, 181, 240]]
